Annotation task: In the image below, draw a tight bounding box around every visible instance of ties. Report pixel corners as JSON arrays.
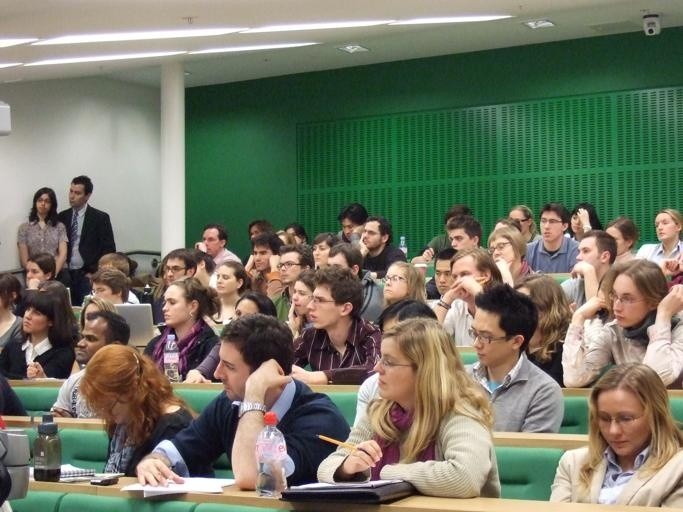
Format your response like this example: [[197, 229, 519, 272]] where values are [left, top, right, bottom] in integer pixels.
[[68, 212, 78, 263]]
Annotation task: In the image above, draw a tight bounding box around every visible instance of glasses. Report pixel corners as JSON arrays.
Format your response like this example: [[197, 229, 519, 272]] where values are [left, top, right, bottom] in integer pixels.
[[277, 261, 302, 270], [381, 275, 405, 283], [610, 295, 645, 303], [488, 219, 563, 255], [308, 296, 335, 304], [469, 326, 506, 344], [375, 353, 412, 371], [593, 414, 644, 426], [163, 267, 186, 273]]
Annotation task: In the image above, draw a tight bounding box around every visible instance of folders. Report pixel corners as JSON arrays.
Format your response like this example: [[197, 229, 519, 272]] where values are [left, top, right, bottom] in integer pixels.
[[278, 481, 417, 503]]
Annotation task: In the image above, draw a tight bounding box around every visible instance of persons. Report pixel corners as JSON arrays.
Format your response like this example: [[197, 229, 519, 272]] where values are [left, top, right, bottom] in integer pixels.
[[1, 202, 682, 511], [59, 175, 118, 308], [16, 186, 68, 280]]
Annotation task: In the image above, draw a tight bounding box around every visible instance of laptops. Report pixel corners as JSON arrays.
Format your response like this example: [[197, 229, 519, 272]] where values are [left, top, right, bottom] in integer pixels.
[[112, 304, 155, 347]]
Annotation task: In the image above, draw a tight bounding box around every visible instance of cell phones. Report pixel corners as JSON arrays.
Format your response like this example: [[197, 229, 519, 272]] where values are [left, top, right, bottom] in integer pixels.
[[90, 477, 119, 485]]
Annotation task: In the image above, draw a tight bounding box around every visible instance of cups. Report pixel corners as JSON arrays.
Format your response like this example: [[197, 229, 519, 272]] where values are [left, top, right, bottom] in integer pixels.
[[414, 263, 426, 279]]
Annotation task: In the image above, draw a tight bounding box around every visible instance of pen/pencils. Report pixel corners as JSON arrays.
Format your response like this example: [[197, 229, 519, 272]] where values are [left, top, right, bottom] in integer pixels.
[[318, 434, 356, 451]]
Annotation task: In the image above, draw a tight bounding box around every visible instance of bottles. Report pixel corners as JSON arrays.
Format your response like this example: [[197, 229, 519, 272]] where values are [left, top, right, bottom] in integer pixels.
[[399, 236, 407, 259], [256, 412, 287, 497], [34, 414, 61, 481], [163, 335, 179, 382], [142, 284, 155, 323]]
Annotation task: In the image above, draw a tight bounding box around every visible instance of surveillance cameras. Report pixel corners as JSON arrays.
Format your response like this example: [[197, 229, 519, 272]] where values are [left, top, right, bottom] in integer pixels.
[[643, 17, 661, 36]]
